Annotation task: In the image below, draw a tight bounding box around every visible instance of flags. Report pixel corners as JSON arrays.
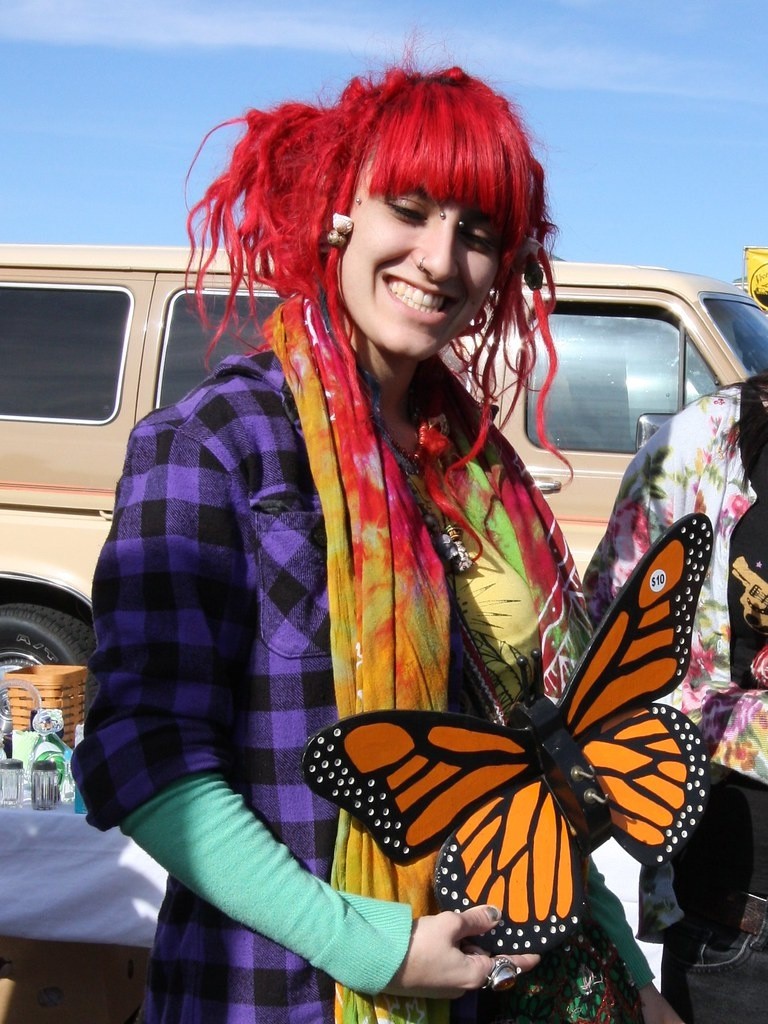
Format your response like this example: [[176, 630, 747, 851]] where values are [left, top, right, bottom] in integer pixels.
[[744, 248, 768, 312]]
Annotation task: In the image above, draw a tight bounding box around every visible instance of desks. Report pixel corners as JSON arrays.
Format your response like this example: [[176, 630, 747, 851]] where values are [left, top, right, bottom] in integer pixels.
[[0, 725, 174, 1024]]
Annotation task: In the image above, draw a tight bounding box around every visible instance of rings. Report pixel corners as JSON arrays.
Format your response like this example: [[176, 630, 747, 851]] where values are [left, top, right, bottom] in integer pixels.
[[483, 963, 521, 992]]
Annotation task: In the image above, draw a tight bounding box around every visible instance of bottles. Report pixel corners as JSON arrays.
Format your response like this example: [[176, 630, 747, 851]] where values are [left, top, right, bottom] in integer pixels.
[[69, 723, 96, 815]]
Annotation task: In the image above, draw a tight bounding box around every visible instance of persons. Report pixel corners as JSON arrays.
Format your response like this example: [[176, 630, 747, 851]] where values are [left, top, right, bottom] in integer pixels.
[[69, 68, 692, 1024], [580, 367, 768, 1024]]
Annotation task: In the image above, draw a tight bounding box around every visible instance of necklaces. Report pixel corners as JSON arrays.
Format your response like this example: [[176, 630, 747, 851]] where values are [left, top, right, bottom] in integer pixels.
[[378, 388, 475, 577]]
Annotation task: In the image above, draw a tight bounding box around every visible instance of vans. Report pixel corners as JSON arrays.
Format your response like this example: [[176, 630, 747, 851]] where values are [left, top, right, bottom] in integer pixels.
[[0, 241, 767, 760]]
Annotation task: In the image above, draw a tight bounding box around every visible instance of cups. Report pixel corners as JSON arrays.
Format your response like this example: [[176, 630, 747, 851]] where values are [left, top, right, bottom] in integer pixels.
[[0, 758, 25, 806], [30, 761, 57, 809]]
